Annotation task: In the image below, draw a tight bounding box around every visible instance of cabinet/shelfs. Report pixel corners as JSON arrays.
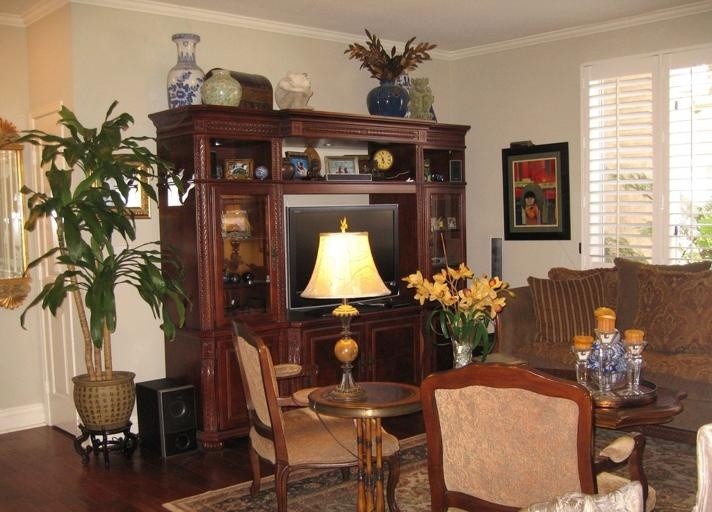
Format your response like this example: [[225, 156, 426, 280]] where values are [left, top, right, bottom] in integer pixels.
[[277, 134, 423, 193], [418, 146, 467, 280], [156, 117, 287, 332], [165, 305, 453, 438]]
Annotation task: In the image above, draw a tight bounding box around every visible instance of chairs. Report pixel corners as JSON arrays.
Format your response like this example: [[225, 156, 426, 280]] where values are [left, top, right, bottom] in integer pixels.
[[232, 326, 401, 512], [420, 362, 656, 512]]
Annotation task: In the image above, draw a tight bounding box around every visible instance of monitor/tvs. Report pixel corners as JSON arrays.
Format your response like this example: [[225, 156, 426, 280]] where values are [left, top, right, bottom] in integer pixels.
[[286, 203, 400, 318]]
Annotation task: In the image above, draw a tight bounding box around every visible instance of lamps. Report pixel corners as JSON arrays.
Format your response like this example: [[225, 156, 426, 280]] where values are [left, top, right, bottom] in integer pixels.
[[300, 231, 392, 404]]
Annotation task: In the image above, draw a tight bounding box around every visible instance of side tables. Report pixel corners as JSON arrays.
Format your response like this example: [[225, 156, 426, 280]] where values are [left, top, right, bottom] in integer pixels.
[[307, 382, 422, 512]]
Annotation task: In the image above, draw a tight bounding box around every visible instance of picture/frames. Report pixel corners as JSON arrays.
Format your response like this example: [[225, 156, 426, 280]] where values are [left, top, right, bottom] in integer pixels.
[[86, 161, 151, 219], [502, 141, 572, 241]]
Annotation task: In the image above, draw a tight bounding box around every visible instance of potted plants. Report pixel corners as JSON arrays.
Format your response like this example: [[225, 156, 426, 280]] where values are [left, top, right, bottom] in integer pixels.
[[0, 99, 195, 431], [343, 28, 437, 118]]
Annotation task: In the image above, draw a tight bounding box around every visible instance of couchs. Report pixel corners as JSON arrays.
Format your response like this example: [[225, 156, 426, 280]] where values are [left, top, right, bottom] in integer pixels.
[[492, 257, 712, 432]]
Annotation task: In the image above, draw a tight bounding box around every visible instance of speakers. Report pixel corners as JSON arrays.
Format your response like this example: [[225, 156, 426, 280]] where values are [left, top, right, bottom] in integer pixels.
[[135, 377, 199, 458], [210, 151, 217, 178], [449, 160, 462, 183]]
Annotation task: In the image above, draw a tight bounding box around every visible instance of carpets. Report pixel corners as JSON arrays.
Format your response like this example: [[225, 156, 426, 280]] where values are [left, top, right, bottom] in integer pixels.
[[160, 428, 698, 511]]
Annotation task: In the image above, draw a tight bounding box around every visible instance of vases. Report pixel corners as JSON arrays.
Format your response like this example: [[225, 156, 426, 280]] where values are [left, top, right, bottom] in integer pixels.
[[451, 337, 473, 368], [166, 33, 206, 109]]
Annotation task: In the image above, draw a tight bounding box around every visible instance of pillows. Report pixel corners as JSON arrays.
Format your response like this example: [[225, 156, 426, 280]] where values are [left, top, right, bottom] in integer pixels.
[[527, 272, 608, 342], [613, 259, 712, 330], [548, 266, 618, 310], [632, 266, 712, 354]]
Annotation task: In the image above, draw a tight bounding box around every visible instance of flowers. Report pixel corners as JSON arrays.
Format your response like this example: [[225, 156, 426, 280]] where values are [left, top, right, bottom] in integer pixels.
[[401, 232, 517, 362]]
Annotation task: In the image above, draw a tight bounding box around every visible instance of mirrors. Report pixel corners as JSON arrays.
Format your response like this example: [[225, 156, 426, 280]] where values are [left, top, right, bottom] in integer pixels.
[[0, 116, 32, 310]]
